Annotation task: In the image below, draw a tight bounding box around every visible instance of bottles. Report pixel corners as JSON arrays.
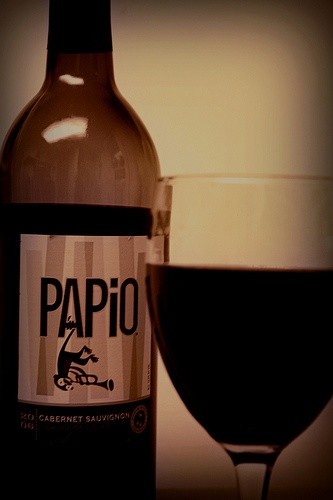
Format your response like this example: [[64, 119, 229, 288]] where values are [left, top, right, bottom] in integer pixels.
[[1, 1, 161, 500]]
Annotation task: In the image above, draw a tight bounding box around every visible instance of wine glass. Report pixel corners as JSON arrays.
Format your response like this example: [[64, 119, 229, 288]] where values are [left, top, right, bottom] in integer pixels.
[[143, 173, 333, 500]]
